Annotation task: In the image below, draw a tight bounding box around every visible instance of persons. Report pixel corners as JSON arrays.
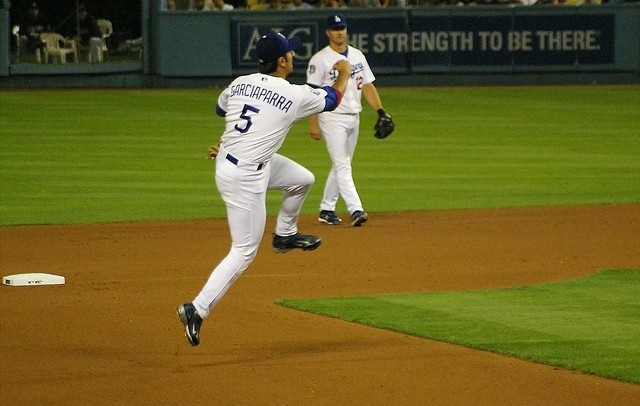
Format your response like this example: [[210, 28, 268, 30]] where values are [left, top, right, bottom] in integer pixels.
[[175, 31, 353, 348], [304, 14, 396, 226]]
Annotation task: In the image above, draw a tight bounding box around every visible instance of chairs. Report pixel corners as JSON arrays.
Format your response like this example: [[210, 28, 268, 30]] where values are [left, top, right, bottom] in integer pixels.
[[40, 32, 78, 64]]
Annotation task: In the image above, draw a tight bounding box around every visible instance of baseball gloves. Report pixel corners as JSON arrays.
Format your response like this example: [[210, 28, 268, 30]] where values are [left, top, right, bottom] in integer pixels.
[[375, 108, 394, 139]]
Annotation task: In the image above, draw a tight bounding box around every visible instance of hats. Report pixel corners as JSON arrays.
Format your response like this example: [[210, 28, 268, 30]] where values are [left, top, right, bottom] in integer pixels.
[[328, 15, 348, 29], [257, 33, 300, 65]]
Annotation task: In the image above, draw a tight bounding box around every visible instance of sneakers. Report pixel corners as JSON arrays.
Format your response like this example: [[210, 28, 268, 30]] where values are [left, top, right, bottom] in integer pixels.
[[176, 304, 204, 347], [352, 211, 368, 227], [272, 233, 322, 254], [318, 211, 343, 226]]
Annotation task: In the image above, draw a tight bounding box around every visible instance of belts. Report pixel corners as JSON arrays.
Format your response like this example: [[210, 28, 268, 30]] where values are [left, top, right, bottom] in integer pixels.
[[341, 114, 358, 116], [227, 154, 264, 171]]
